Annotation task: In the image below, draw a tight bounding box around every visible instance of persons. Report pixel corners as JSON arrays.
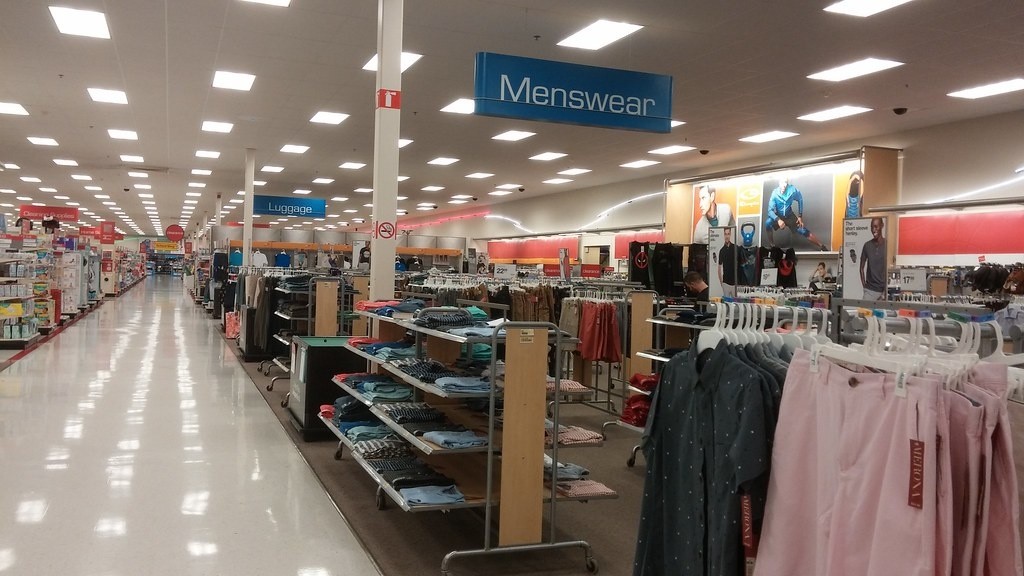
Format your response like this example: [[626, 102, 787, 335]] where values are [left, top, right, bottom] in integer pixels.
[[560, 249, 570, 281], [685, 271, 710, 315], [477, 252, 485, 273], [356, 240, 371, 268], [765, 178, 828, 252], [693, 185, 736, 251], [845, 176, 862, 218], [717, 228, 736, 298], [808, 262, 833, 294], [859, 218, 886, 300]]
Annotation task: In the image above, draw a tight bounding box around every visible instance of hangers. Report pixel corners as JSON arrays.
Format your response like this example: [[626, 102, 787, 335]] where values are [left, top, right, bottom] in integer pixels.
[[223, 248, 1024, 402]]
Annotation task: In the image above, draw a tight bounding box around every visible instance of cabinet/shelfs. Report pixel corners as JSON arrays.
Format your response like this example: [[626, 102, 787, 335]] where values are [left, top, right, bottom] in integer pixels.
[[190, 249, 660, 576], [0, 246, 184, 351]]
